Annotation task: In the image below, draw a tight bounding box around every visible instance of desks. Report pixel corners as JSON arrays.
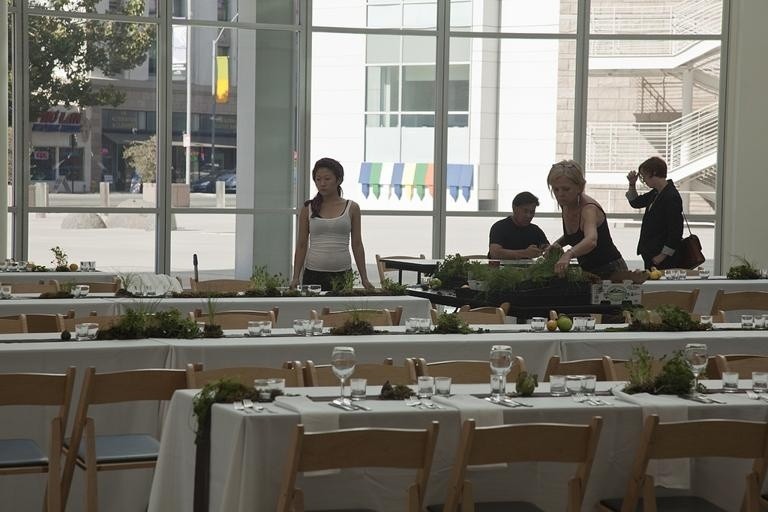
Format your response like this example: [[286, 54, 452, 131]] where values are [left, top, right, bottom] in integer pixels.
[[148, 376, 767, 512], [0, 295, 433, 333], [0, 269, 117, 299], [379, 259, 766, 322], [0, 329, 768, 512]]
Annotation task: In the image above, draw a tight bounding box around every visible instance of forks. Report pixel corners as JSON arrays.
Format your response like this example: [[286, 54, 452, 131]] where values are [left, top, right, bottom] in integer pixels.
[[570, 390, 614, 406], [746, 390, 768, 402], [403, 395, 444, 410], [233, 399, 276, 415]]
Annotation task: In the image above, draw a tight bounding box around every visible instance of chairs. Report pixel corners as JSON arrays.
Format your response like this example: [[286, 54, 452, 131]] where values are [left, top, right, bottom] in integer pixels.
[[58, 277, 123, 294], [61, 360, 204, 510], [3, 278, 60, 294], [2, 366, 77, 511], [641, 288, 701, 315], [541, 355, 636, 382], [373, 253, 426, 285], [430, 307, 508, 325], [188, 310, 275, 334], [0, 314, 29, 334], [187, 357, 305, 393], [309, 309, 395, 327], [455, 254, 489, 260], [700, 352, 768, 379], [410, 353, 526, 384], [194, 306, 280, 322], [322, 306, 405, 330], [12, 309, 175, 333], [710, 290, 766, 321], [283, 356, 417, 388], [190, 276, 257, 295], [459, 300, 509, 317]]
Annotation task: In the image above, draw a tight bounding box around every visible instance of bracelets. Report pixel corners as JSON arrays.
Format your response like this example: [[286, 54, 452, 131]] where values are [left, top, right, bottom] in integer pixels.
[[567, 246, 578, 260]]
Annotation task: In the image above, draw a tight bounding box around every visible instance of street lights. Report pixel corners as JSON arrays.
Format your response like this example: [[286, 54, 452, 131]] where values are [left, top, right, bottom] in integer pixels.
[[130, 126, 138, 194], [67, 133, 77, 194]]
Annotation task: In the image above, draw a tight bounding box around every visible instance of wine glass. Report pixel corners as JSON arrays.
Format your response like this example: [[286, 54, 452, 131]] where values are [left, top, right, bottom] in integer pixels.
[[333, 346, 356, 404], [686, 344, 708, 396], [490, 344, 513, 401]]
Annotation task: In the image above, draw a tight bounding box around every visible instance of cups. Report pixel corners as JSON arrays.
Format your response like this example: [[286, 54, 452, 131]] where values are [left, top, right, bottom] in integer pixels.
[[72, 285, 90, 296], [350, 379, 366, 400], [74, 323, 99, 341], [530, 317, 546, 330], [700, 315, 712, 330], [80, 260, 95, 273], [298, 283, 321, 295], [248, 321, 271, 336], [722, 371, 739, 388], [541, 244, 549, 259], [405, 318, 431, 333], [751, 372, 768, 393], [665, 270, 711, 280], [293, 319, 324, 335], [741, 314, 768, 329], [436, 377, 452, 396], [490, 375, 506, 396], [421, 277, 432, 290], [0, 284, 12, 298], [574, 317, 597, 331], [416, 376, 434, 397], [549, 374, 567, 395], [254, 378, 285, 394], [133, 285, 156, 296], [580, 374, 597, 394]]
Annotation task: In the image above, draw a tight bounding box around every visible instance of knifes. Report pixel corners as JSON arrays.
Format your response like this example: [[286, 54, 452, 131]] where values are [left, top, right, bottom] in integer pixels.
[[330, 398, 371, 411], [679, 393, 725, 405], [485, 396, 532, 407]]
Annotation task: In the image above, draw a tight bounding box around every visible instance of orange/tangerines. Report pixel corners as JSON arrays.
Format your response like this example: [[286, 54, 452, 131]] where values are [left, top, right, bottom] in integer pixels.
[[557, 317, 573, 331]]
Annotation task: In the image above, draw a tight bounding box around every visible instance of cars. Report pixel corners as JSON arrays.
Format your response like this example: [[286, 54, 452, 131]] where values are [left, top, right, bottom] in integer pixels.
[[189, 163, 236, 194]]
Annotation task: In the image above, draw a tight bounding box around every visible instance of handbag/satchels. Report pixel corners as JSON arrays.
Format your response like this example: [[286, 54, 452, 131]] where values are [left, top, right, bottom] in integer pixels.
[[680, 234, 706, 270]]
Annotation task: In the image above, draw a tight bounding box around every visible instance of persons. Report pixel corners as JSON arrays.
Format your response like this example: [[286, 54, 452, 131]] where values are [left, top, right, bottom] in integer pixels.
[[625, 156, 684, 273], [541, 160, 628, 279], [288, 157, 374, 291], [484, 191, 550, 259]]
